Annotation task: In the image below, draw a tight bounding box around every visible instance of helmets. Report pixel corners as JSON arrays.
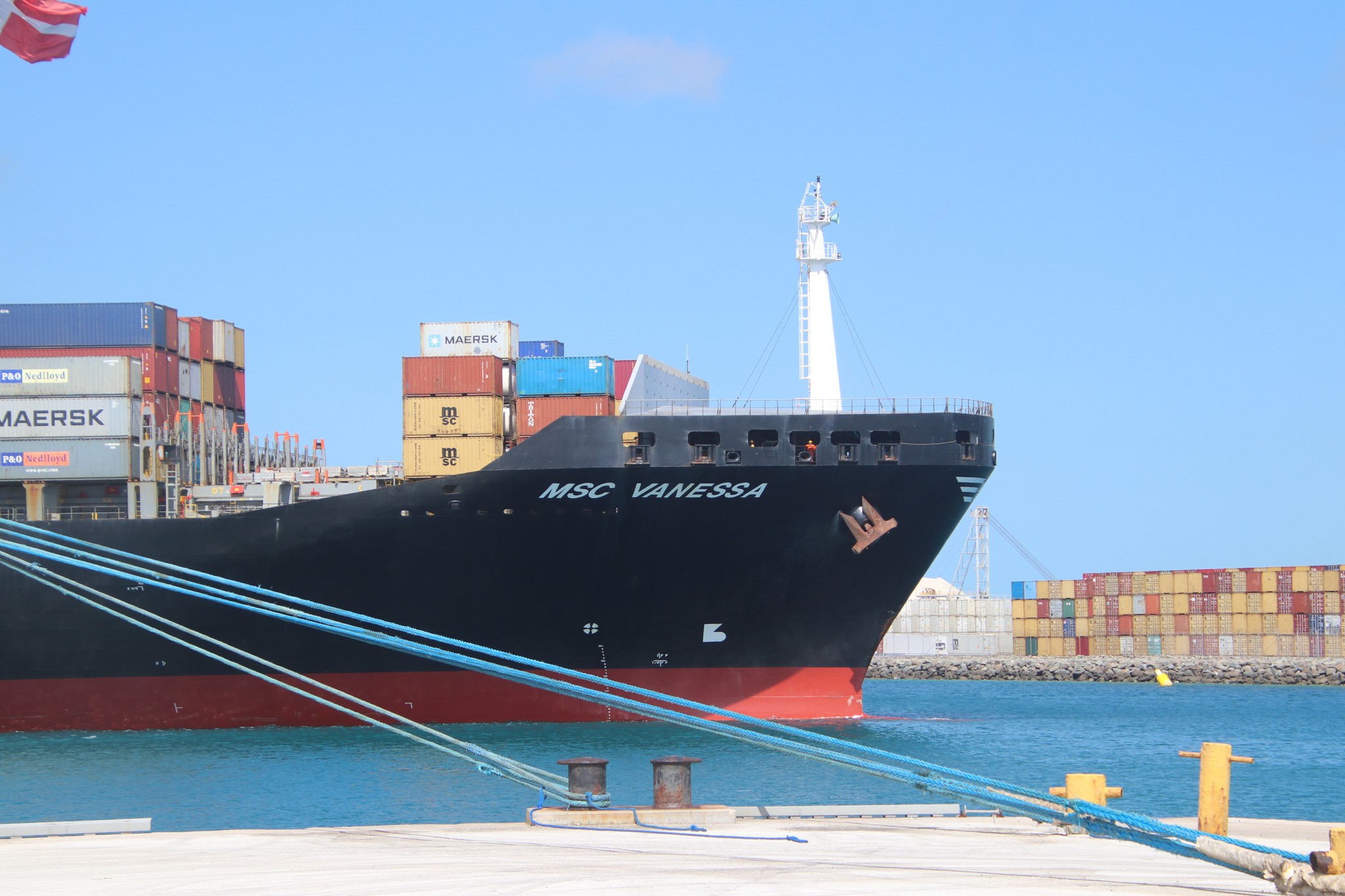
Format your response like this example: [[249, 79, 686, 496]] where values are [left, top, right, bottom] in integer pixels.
[[750, 440, 754, 443], [808, 440, 812, 443]]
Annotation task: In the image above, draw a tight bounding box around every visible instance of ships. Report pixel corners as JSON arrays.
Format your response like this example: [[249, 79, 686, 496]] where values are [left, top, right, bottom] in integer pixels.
[[0, 174, 998, 734]]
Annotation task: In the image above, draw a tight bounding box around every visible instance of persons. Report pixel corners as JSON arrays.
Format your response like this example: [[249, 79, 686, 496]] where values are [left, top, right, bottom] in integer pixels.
[[805, 440, 816, 461], [749, 440, 754, 447]]
